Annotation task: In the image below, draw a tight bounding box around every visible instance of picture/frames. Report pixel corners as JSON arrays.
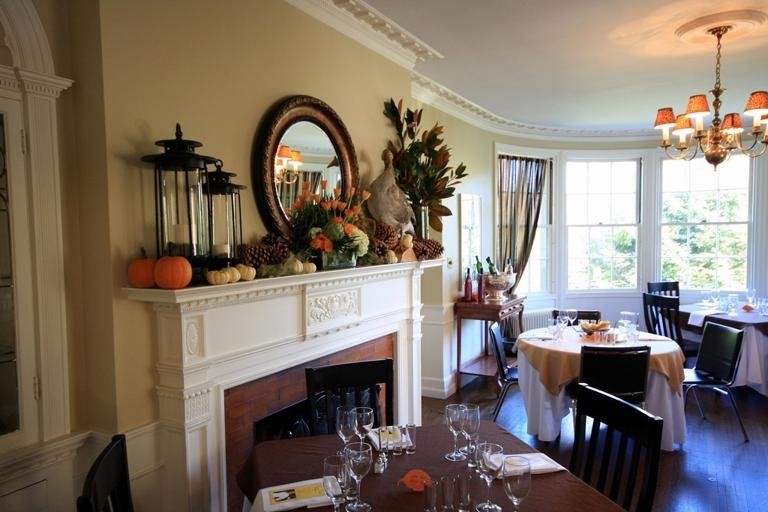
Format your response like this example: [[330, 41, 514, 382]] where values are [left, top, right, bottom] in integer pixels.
[[458, 192, 483, 291]]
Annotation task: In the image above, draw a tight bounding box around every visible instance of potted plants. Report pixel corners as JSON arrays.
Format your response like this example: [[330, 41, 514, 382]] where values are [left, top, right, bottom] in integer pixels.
[[379, 99, 468, 242]]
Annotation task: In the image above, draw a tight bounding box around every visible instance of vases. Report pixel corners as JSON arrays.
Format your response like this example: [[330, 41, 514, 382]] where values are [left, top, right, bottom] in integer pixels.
[[322, 247, 357, 269]]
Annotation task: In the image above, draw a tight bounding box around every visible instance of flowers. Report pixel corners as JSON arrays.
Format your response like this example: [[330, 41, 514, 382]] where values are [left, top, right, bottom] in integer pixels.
[[307, 186, 375, 257], [286, 177, 340, 219]]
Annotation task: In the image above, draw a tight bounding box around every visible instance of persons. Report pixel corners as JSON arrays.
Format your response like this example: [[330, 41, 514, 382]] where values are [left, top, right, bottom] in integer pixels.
[[273, 490, 295, 501]]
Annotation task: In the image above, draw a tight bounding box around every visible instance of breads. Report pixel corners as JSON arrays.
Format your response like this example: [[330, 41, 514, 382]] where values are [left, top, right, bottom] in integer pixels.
[[581, 321, 609, 330]]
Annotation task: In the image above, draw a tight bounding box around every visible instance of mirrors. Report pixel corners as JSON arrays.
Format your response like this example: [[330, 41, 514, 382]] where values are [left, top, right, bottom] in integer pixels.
[[249, 92, 362, 235]]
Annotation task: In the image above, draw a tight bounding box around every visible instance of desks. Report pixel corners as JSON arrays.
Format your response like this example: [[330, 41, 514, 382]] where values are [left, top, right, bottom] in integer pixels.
[[455, 295, 528, 407]]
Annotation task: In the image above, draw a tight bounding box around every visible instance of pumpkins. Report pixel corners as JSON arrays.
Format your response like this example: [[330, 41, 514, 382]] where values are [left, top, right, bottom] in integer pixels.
[[288, 258, 317, 274], [154, 242, 193, 289], [401, 234, 413, 252], [384, 250, 398, 263], [126, 247, 155, 288], [206, 261, 257, 285]]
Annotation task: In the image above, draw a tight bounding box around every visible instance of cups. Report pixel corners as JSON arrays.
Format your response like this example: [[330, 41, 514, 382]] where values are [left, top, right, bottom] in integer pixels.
[[378, 428, 389, 456], [702, 289, 768, 316], [422, 479, 438, 512], [392, 425, 403, 456], [593, 330, 614, 344], [439, 474, 456, 512], [406, 424, 416, 455], [614, 319, 638, 343], [456, 472, 473, 512]]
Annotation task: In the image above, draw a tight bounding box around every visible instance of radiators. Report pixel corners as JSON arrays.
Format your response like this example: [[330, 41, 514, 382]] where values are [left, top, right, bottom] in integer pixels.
[[506, 307, 560, 344]]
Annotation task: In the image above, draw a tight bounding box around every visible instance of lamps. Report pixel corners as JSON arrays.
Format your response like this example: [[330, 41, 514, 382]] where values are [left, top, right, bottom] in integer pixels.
[[652, 9, 768, 172], [274, 146, 303, 186]]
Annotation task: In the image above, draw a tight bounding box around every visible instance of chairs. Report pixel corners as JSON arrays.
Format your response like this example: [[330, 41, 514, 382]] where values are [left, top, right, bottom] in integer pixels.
[[567, 380, 664, 512], [76, 432, 136, 512], [304, 357, 394, 441], [565, 345, 653, 447], [554, 310, 601, 326], [682, 322, 751, 444], [646, 281, 700, 358], [642, 292, 687, 370], [488, 321, 521, 424]]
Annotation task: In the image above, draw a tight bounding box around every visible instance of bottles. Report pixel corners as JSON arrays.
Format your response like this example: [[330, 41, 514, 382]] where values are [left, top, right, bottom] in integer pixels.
[[474, 256, 483, 274], [465, 268, 472, 302], [485, 256, 499, 275], [374, 452, 388, 474], [505, 258, 513, 274], [477, 268, 485, 303]]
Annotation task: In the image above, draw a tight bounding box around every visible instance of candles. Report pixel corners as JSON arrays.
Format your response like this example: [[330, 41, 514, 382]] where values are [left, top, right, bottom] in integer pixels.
[[172, 223, 197, 246], [214, 244, 231, 258]]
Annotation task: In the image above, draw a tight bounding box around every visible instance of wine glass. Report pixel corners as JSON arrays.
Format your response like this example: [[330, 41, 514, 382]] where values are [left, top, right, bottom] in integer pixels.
[[444, 403, 466, 462], [344, 442, 373, 512], [474, 442, 504, 512], [502, 456, 532, 512], [322, 453, 351, 511], [556, 309, 578, 335], [459, 403, 480, 452], [335, 405, 357, 456], [351, 407, 375, 465], [481, 270, 518, 302]]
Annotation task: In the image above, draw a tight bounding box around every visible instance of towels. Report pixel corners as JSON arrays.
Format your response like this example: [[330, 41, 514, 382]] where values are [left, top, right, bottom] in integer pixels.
[[250, 474, 345, 512], [489, 451, 570, 482], [367, 426, 404, 452]]
